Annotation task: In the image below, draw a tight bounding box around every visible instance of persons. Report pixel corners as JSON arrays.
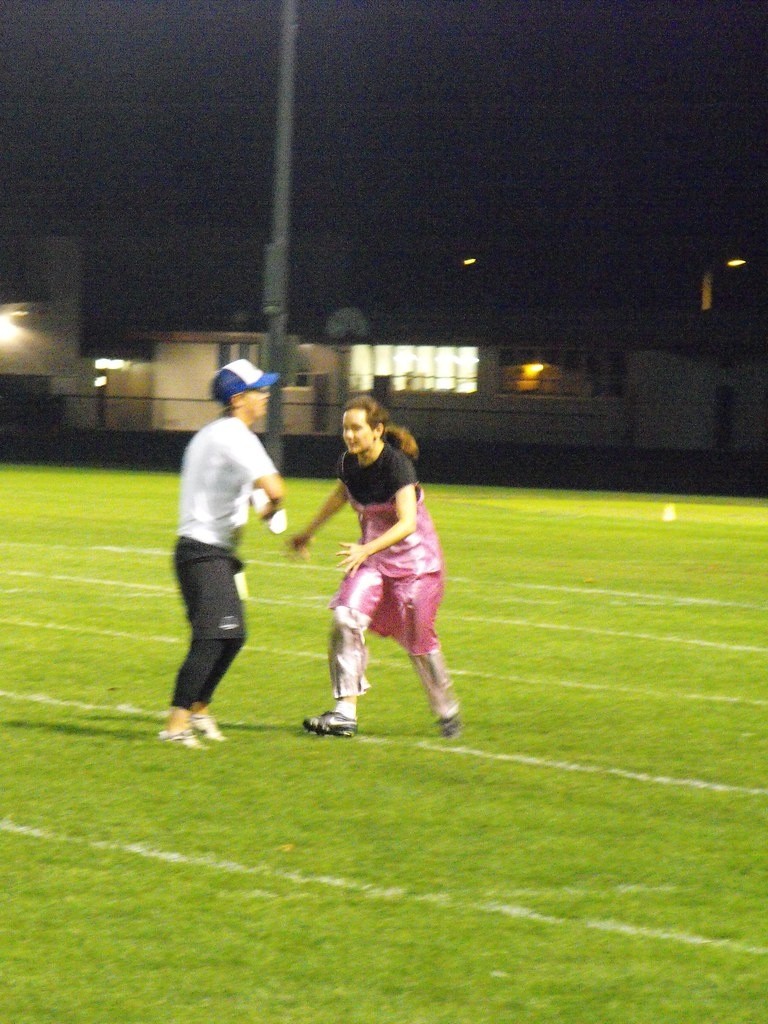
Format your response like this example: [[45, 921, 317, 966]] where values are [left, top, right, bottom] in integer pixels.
[[154, 359, 286, 749], [287, 394, 459, 738]]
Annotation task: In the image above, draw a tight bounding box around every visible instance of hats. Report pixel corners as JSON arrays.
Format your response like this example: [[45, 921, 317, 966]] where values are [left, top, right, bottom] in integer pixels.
[[214, 358, 281, 401]]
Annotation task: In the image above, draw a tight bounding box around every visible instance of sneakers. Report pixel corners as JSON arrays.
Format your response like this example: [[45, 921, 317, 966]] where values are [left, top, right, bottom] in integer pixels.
[[160, 729, 204, 750], [193, 714, 225, 741], [304, 711, 358, 736], [441, 718, 458, 736]]
